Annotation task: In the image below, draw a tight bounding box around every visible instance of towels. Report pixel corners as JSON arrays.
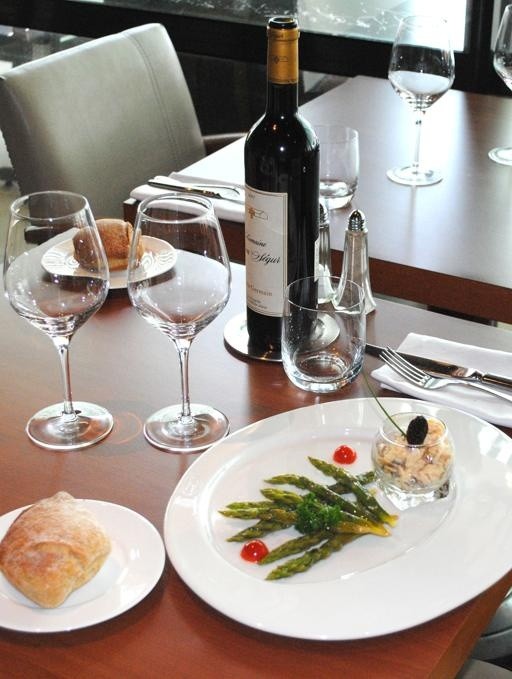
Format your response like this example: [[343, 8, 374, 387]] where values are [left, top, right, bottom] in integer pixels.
[[371, 333, 512, 429]]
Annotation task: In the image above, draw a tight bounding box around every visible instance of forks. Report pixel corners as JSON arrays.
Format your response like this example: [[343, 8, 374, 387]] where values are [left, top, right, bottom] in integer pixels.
[[377, 344, 511, 405]]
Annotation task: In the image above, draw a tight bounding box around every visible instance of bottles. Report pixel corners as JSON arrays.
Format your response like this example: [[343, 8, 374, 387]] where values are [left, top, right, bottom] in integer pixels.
[[317, 202, 334, 306], [330, 209, 377, 318], [241, 15, 318, 350]]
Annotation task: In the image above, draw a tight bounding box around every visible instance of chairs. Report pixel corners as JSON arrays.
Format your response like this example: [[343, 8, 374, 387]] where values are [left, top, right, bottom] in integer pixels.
[[0, 23, 246, 241]]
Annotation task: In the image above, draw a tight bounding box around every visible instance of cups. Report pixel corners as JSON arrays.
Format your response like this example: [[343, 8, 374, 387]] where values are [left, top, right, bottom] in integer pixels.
[[310, 124, 361, 212], [278, 275, 368, 397], [370, 412, 455, 511]]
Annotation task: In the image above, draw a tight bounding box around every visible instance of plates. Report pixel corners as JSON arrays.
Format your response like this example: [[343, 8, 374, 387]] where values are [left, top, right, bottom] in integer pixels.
[[38, 229, 178, 293], [162, 396, 511, 645], [1, 500, 168, 637]]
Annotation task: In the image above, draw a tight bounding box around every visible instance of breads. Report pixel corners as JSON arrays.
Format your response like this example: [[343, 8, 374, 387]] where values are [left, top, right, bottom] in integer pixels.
[[0, 490, 114, 610], [70, 219, 144, 273]]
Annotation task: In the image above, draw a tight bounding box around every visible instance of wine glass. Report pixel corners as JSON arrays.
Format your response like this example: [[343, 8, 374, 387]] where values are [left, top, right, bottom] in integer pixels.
[[4, 191, 114, 453], [124, 192, 237, 455], [385, 15, 456, 186], [488, 4, 511, 167]]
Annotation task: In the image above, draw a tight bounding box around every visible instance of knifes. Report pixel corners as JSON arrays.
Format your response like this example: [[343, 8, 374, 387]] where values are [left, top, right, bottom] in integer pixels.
[[145, 173, 244, 205], [348, 336, 511, 389]]
